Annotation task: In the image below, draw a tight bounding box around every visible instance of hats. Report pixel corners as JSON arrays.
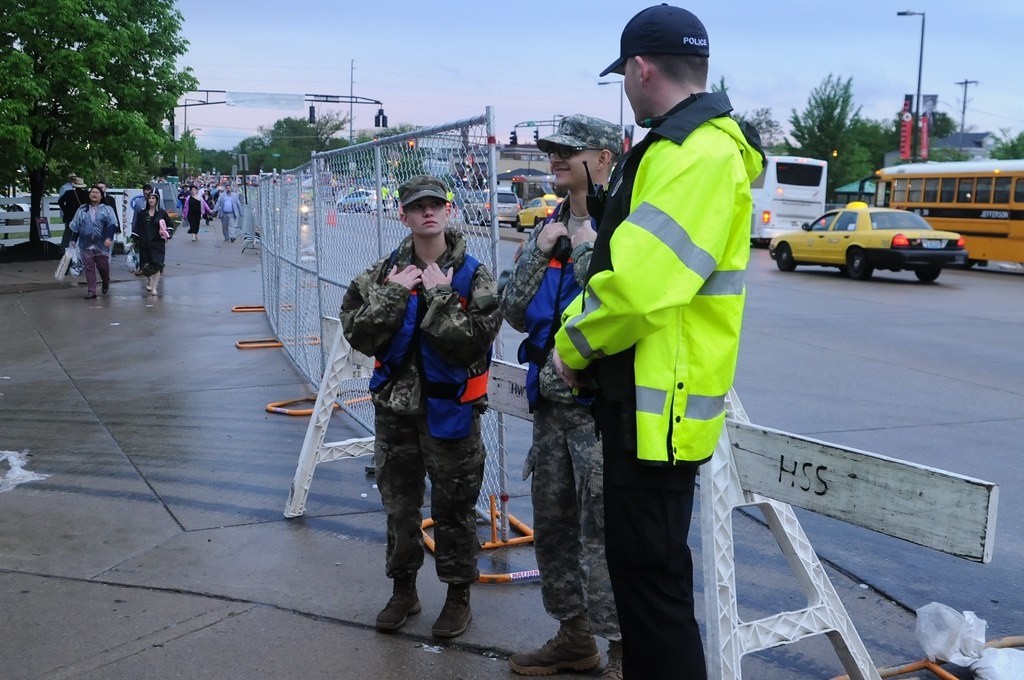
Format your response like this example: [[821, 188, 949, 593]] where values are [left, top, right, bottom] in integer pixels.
[[183, 184, 188, 187], [599, 3, 709, 77], [204, 189, 210, 192], [72, 177, 87, 188], [63, 173, 77, 181], [398, 175, 449, 206], [537, 113, 623, 155]]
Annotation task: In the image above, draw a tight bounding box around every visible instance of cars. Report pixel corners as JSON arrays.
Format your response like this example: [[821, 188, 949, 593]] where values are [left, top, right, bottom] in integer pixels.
[[336, 190, 396, 213], [516, 194, 567, 233], [768, 200, 970, 284]]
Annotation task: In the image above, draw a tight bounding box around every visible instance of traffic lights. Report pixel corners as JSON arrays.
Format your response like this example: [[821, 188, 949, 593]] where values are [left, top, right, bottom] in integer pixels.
[[374, 115, 380, 127], [534, 130, 539, 142], [509, 131, 518, 145]]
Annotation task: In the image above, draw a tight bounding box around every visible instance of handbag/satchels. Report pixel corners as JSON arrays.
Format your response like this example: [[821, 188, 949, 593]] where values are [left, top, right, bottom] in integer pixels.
[[181, 217, 190, 227], [126, 248, 140, 273], [55, 247, 85, 282]]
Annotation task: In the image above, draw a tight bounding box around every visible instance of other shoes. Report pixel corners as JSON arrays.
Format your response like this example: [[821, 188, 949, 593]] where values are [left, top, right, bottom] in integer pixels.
[[146, 285, 151, 292], [195, 236, 198, 241], [191, 236, 196, 241], [151, 289, 158, 296], [230, 237, 236, 243], [102, 284, 109, 294], [84, 292, 97, 300], [225, 235, 229, 243]]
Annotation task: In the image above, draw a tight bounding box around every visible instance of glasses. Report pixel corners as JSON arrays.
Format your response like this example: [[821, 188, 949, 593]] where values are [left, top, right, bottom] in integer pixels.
[[548, 145, 603, 159]]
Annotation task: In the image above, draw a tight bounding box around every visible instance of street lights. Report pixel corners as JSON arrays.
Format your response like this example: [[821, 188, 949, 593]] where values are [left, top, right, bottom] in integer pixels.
[[896, 10, 926, 166], [597, 80, 625, 132], [183, 99, 206, 185]]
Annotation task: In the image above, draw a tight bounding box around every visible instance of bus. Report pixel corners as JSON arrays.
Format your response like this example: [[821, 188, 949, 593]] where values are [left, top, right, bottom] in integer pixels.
[[857, 159, 1024, 266], [511, 175, 571, 207], [749, 155, 828, 247]]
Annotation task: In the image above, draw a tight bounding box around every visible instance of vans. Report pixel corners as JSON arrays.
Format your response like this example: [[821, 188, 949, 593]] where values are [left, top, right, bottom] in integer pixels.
[[463, 189, 522, 227]]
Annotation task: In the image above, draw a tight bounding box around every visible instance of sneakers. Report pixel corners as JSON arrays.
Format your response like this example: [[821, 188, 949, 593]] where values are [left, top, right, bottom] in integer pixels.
[[375, 577, 422, 631], [508, 613, 601, 676], [431, 584, 473, 638], [599, 640, 623, 680]]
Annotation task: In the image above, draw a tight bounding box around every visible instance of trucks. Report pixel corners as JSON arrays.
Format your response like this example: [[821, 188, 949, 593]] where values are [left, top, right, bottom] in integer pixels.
[[105, 188, 166, 243]]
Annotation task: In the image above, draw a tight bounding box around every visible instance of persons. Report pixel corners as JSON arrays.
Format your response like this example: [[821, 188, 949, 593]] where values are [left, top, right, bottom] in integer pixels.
[[552, 4, 766, 680], [129, 184, 174, 295], [331, 174, 400, 209], [338, 177, 505, 637], [150, 175, 168, 184], [178, 174, 244, 241], [446, 187, 454, 208], [501, 114, 624, 680], [59, 169, 121, 299]]
